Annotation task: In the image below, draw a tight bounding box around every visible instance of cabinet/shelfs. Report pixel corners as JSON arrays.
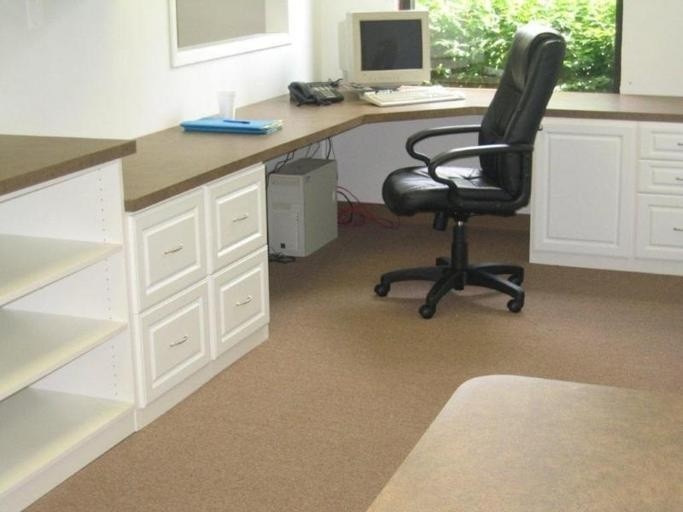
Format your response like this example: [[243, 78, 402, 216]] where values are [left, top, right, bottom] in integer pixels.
[[0, 157, 136, 511], [638, 120, 683, 261], [124, 163, 271, 408], [528, 116, 638, 259]]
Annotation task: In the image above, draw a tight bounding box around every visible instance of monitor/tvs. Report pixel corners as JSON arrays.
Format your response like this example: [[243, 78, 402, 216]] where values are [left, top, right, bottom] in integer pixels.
[[337, 11, 431, 91]]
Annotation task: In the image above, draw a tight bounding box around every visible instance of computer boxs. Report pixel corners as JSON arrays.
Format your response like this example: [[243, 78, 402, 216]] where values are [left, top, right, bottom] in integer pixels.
[[267, 158, 338, 258]]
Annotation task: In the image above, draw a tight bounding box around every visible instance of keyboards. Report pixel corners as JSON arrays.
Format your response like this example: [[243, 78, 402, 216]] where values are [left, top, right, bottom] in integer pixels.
[[359, 85, 467, 107]]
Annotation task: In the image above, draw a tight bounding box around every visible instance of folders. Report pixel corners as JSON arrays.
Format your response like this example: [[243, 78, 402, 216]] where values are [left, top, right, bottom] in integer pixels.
[[180, 116, 284, 135]]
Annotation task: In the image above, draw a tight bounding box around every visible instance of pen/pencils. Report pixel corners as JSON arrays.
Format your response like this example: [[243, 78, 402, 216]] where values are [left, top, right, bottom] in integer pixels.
[[223, 120, 250, 124]]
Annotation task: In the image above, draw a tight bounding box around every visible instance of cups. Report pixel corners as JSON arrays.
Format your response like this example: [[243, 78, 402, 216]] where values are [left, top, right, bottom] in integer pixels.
[[216, 88, 237, 120]]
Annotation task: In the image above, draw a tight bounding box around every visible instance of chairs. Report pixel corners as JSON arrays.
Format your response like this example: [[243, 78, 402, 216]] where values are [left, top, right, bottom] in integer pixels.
[[373, 20, 566, 319]]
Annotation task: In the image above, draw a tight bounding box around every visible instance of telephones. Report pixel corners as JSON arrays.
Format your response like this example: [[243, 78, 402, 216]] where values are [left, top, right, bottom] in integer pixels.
[[288, 82, 344, 107]]
